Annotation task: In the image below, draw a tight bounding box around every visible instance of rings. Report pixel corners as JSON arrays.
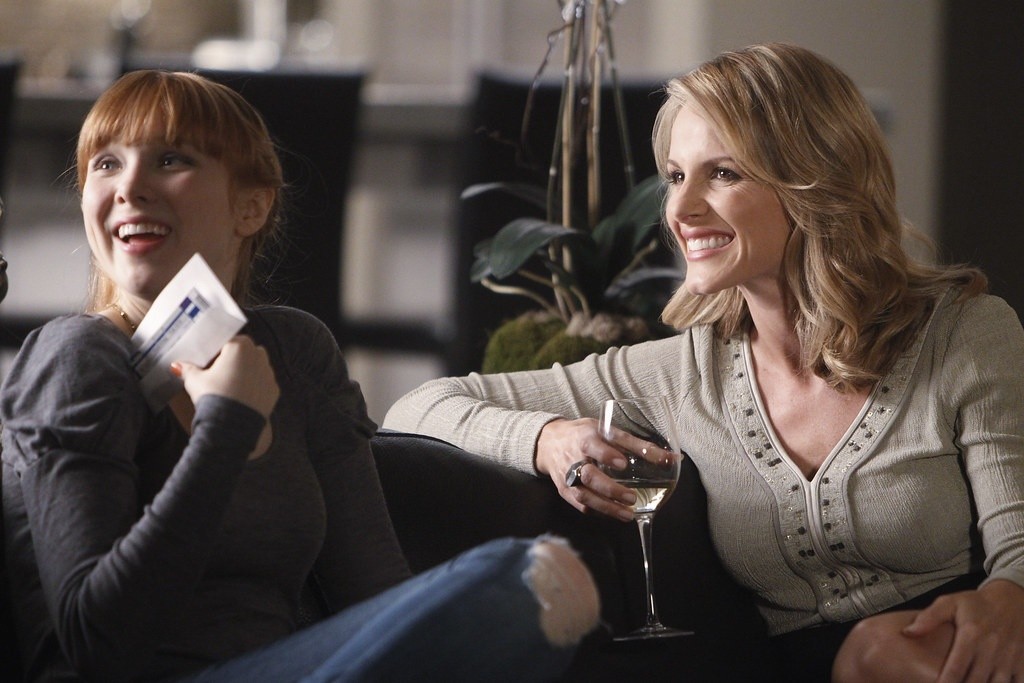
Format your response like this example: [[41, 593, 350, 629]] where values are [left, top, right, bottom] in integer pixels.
[[566, 459, 589, 486]]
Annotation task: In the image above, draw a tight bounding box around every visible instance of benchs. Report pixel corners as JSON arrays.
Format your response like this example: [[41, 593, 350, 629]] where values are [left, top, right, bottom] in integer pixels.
[[1, 429, 772, 683]]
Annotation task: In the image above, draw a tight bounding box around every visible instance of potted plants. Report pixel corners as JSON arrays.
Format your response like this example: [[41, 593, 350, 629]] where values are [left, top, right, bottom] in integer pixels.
[[458, 179, 677, 375]]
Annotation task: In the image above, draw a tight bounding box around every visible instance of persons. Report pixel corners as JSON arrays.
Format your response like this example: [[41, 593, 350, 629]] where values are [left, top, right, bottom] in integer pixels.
[[382, 42, 1024, 683], [0, 69, 599, 683]]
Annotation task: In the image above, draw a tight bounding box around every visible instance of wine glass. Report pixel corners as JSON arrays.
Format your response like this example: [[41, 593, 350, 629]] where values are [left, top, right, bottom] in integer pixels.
[[597, 395, 694, 641]]
